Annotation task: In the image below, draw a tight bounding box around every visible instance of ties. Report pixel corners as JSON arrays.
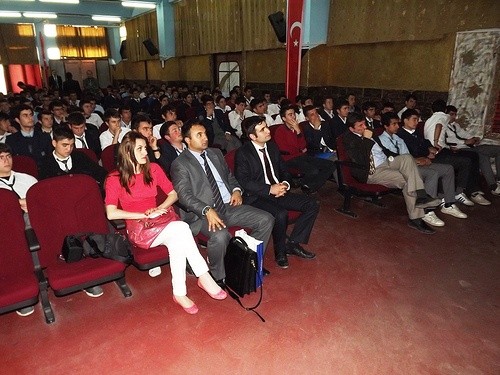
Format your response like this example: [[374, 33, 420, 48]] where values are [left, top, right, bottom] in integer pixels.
[[368, 121, 372, 128], [389, 126, 468, 154], [0, 158, 69, 199], [259, 148, 276, 185], [200, 152, 226, 214], [77, 137, 87, 148]]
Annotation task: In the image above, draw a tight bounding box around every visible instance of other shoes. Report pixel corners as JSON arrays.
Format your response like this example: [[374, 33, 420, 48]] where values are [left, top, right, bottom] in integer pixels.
[[491, 186, 500, 195], [174, 295, 198, 314], [496, 181, 500, 186], [300, 184, 310, 191], [198, 278, 227, 300], [262, 268, 270, 276]]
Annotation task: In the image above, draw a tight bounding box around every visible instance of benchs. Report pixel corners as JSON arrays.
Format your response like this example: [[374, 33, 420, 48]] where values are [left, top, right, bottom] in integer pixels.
[[0, 107, 425, 323]]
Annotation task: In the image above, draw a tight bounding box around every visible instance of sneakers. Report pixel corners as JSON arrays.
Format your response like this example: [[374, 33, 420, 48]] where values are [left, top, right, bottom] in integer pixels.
[[441, 204, 468, 218], [422, 211, 444, 226], [415, 194, 441, 208], [16, 306, 34, 316], [83, 286, 104, 298], [471, 194, 491, 205], [454, 193, 474, 205], [408, 218, 435, 234]]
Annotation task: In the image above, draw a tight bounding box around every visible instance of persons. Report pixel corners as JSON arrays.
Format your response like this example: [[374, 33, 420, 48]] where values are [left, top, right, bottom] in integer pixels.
[[165, 119, 277, 291], [342, 110, 444, 235], [232, 115, 321, 267], [0, 68, 500, 317], [82, 69, 100, 98], [60, 72, 83, 97], [47, 69, 62, 91], [102, 130, 228, 315]]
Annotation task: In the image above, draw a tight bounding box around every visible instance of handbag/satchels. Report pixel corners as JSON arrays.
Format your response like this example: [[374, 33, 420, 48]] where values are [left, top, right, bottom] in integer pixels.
[[62, 236, 83, 262], [235, 229, 264, 287], [224, 238, 257, 297], [84, 234, 130, 263]]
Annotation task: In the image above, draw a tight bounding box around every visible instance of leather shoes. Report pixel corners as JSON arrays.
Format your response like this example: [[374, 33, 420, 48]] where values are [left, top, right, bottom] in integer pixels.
[[286, 243, 314, 258], [275, 248, 288, 268]]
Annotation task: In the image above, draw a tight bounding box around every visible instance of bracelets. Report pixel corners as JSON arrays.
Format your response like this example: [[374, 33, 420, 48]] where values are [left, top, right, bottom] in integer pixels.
[[203, 206, 211, 214]]
